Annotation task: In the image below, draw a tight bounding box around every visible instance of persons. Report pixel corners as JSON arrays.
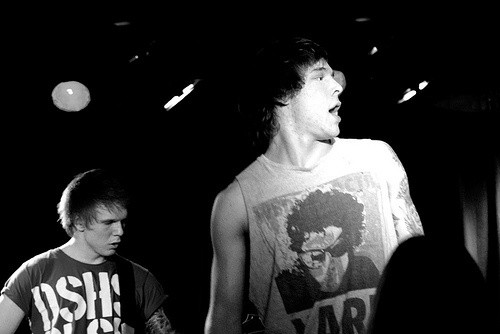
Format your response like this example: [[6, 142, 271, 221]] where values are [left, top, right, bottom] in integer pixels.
[[202, 34, 424, 334], [371, 234, 500, 334], [1, 168, 178, 334]]
